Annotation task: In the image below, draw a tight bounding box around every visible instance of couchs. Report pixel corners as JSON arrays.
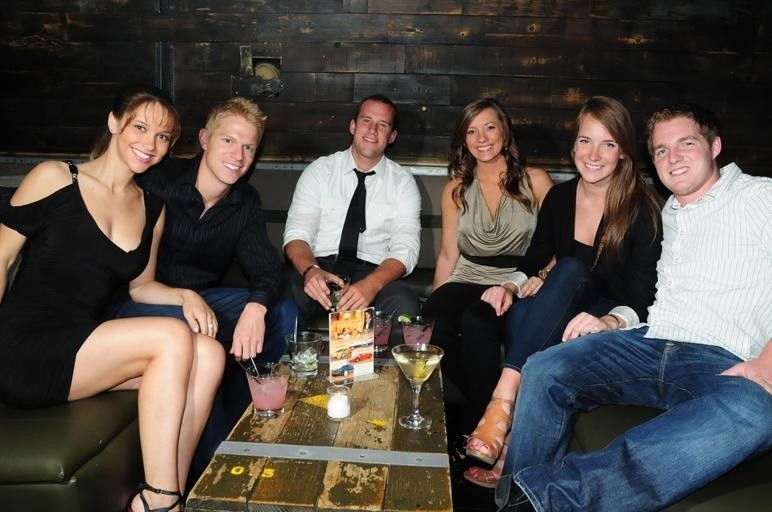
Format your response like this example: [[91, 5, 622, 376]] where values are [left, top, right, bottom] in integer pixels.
[[0, 158, 770, 511]]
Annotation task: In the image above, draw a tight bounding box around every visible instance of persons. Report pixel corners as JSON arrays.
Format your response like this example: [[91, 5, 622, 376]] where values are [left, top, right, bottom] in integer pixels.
[[1, 87, 227, 512], [418, 98, 557, 473], [494, 103, 772, 512], [358, 309, 374, 335], [132, 96, 305, 468], [279, 92, 423, 347], [463, 94, 665, 491]]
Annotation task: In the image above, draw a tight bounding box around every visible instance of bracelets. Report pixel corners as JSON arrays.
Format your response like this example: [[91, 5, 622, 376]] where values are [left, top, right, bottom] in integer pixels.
[[303, 262, 321, 278], [608, 309, 625, 330]]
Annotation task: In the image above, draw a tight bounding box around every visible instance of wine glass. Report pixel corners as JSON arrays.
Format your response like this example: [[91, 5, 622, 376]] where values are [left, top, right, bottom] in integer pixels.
[[391, 342, 444, 430]]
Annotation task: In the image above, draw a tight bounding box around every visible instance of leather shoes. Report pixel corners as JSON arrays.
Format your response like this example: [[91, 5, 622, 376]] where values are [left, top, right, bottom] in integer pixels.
[[493, 471, 538, 512]]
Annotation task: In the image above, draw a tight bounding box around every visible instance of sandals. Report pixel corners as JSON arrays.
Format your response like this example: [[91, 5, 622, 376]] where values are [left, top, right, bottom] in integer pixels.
[[454, 430, 512, 489], [464, 396, 516, 467], [126, 481, 186, 512]]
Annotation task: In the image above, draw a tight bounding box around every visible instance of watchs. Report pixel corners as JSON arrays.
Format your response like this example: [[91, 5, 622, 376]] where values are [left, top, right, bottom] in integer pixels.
[[536, 264, 550, 281]]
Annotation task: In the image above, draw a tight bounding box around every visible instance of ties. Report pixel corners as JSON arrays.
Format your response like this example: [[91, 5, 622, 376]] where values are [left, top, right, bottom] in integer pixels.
[[332, 168, 376, 274]]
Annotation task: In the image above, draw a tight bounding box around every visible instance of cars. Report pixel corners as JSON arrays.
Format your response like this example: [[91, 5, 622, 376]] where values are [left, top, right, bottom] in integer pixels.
[[332, 347, 352, 360], [331, 364, 354, 377], [349, 352, 373, 362]]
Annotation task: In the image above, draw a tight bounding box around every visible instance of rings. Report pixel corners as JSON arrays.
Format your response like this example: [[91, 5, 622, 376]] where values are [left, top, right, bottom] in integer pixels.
[[208, 325, 213, 330]]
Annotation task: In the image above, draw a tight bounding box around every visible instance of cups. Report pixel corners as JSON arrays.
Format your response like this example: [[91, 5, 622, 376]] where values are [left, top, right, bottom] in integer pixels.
[[245, 361, 292, 418], [284, 331, 322, 378], [325, 274, 351, 311], [401, 315, 436, 344], [373, 308, 396, 353]]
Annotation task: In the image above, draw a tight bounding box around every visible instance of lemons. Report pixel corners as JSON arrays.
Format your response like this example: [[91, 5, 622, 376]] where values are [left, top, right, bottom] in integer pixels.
[[397, 314, 412, 324]]
